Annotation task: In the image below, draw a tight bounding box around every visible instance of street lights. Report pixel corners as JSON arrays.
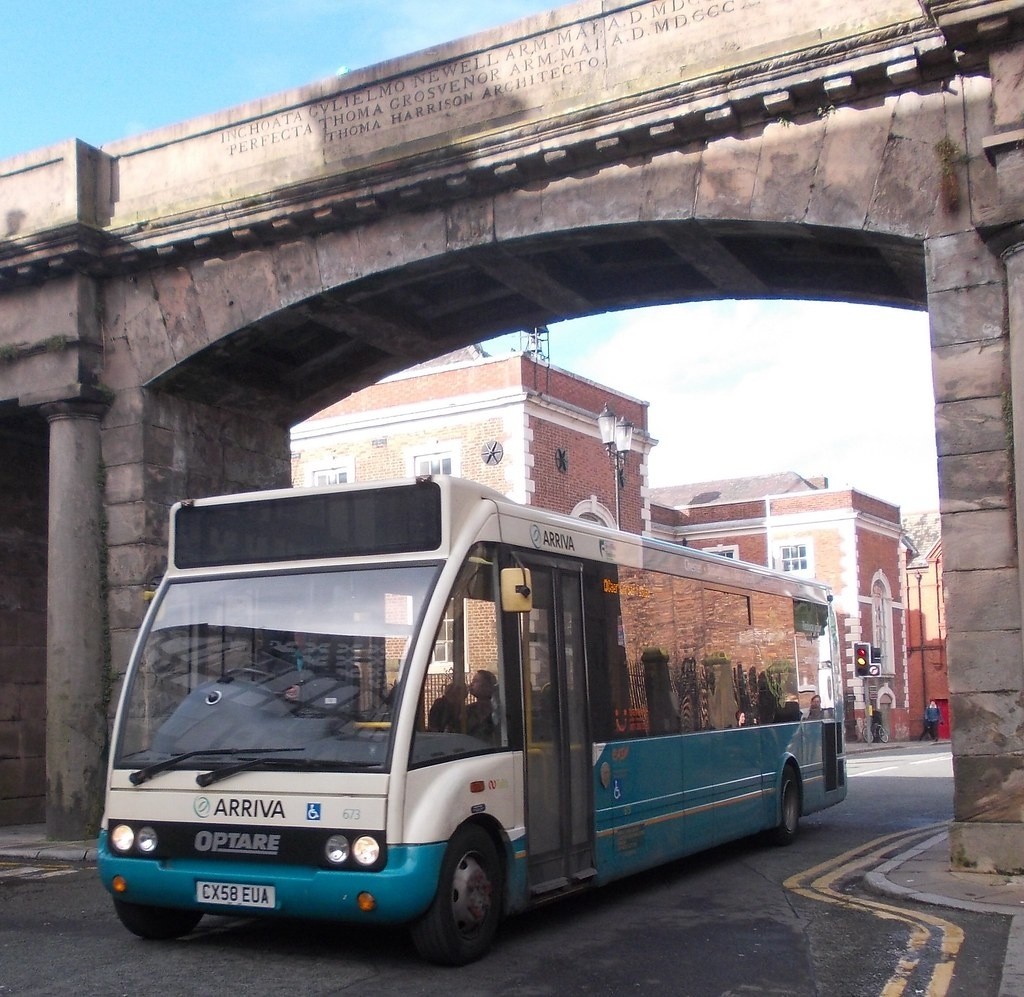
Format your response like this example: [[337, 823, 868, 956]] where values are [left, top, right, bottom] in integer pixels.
[[596, 402, 634, 533]]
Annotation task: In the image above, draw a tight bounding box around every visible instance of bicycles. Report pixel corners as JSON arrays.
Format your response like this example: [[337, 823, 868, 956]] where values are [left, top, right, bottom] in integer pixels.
[[862, 722, 888, 743]]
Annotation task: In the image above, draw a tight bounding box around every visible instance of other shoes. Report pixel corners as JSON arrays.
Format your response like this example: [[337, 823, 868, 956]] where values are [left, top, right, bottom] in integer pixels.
[[932, 738, 938, 742]]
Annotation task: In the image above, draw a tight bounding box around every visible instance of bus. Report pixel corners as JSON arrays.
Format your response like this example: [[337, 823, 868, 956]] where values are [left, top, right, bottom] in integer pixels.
[[97, 474, 848, 964]]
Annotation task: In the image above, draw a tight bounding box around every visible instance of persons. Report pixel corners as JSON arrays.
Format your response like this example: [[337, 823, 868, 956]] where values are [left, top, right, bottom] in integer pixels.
[[798, 693, 829, 766], [924, 699, 943, 742], [918, 706, 936, 742], [267, 615, 347, 721], [733, 709, 751, 730], [432, 662, 504, 742]]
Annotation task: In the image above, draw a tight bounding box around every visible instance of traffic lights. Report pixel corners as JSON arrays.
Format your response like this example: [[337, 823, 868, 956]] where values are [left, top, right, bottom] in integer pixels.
[[853, 643, 871, 678]]
